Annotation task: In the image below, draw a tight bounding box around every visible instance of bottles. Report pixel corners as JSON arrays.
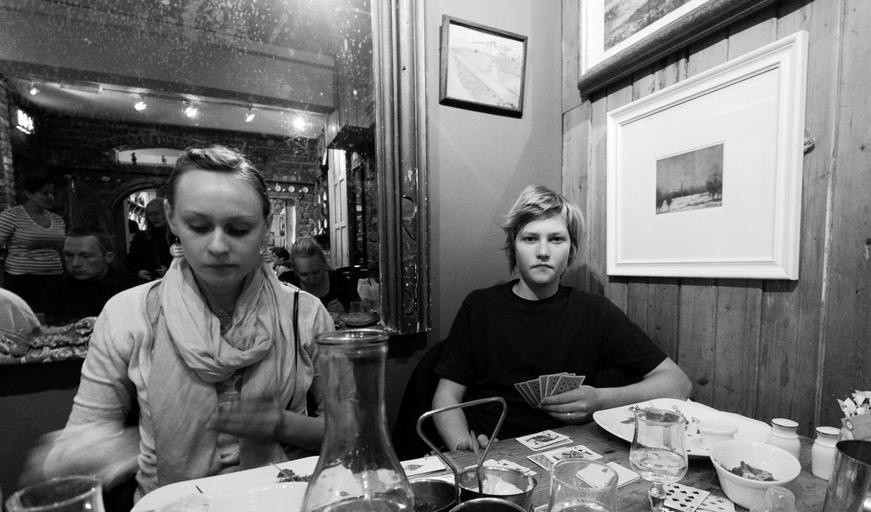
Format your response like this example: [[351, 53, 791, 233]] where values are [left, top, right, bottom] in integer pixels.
[[811, 426, 843, 483], [746, 486, 802, 510], [301, 327, 417, 511], [768, 417, 802, 462]]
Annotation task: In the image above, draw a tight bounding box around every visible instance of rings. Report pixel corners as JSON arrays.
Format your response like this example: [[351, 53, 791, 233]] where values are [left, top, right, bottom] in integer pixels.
[[567, 412, 572, 421]]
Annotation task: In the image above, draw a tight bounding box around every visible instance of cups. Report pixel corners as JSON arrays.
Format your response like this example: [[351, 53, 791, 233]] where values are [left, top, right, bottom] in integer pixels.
[[388, 479, 457, 511], [456, 466, 538, 512], [698, 418, 737, 449], [548, 459, 620, 512], [448, 498, 528, 512], [822, 441, 871, 512], [35, 311, 49, 331], [6, 476, 106, 511], [348, 301, 363, 324]]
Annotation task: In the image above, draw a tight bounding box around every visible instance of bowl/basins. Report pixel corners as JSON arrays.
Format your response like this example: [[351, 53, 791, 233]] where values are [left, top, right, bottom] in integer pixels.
[[710, 438, 802, 510], [327, 311, 373, 323]]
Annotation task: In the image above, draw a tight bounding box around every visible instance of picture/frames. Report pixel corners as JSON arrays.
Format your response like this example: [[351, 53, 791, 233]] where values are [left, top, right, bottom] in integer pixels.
[[441, 12, 527, 118], [576, 0, 830, 99], [602, 29, 809, 284]]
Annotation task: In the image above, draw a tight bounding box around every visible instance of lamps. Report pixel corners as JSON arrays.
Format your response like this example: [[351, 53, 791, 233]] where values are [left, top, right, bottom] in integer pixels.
[[27, 78, 255, 119]]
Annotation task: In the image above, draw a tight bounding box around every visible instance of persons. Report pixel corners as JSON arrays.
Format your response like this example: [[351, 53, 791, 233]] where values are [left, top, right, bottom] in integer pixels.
[[43, 224, 141, 323], [432, 183, 693, 452], [44, 144, 359, 506], [1, 175, 66, 313], [268, 236, 362, 314], [124, 197, 177, 283]]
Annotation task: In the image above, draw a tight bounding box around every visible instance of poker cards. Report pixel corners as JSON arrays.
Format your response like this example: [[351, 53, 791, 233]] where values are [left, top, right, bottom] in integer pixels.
[[661, 483, 735, 512], [513, 372, 585, 408], [464, 459, 536, 476], [575, 462, 640, 489], [515, 430, 572, 452], [399, 456, 446, 479], [526, 445, 602, 471]]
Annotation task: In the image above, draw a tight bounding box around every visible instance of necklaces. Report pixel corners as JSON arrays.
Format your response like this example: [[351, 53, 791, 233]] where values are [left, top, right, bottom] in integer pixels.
[[29, 208, 42, 220], [213, 311, 233, 317]]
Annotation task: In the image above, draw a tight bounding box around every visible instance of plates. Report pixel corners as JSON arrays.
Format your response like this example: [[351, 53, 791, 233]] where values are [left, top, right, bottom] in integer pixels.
[[593, 396, 773, 458], [126, 449, 364, 511]]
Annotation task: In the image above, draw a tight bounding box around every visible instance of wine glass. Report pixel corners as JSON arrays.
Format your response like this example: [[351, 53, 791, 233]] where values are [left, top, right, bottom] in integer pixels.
[[628, 407, 691, 498]]
